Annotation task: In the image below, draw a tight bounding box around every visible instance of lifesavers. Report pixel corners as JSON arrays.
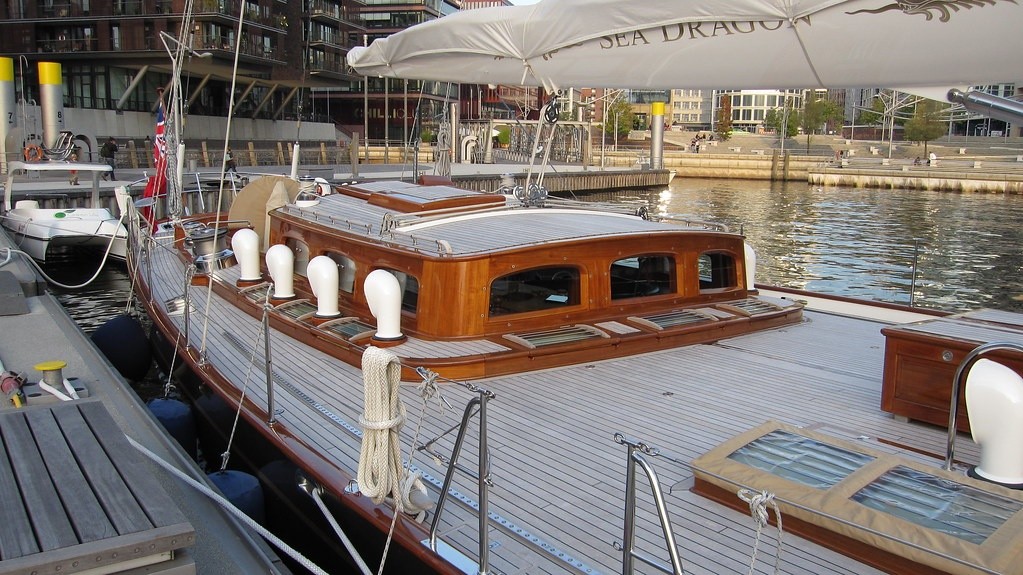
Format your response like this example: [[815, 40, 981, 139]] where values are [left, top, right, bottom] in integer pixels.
[[25, 144, 42, 162]]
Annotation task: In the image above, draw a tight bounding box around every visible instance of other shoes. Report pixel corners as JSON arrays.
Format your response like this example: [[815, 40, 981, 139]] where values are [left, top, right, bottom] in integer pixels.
[[102, 176, 107, 181], [74, 184, 79, 185], [111, 179, 118, 181]]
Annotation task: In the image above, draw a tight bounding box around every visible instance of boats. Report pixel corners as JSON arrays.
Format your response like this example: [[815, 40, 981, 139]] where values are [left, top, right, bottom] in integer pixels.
[[0, 57, 132, 266], [124, 0, 1023, 575]]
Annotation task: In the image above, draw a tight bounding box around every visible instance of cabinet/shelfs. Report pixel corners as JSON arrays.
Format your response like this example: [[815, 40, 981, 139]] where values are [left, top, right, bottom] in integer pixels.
[[880, 308, 1023, 434]]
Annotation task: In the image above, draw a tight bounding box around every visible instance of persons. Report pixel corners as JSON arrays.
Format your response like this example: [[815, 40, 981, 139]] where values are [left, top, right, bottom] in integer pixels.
[[927, 151, 937, 165], [66, 153, 81, 186], [690, 133, 714, 154], [913, 155, 921, 167], [834, 160, 835, 160], [101, 137, 119, 181], [834, 149, 846, 170], [558, 287, 569, 302], [220, 146, 241, 179]]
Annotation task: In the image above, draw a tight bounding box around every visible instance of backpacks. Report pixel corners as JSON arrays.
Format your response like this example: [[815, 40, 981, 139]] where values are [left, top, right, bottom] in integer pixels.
[[101, 144, 109, 157]]
